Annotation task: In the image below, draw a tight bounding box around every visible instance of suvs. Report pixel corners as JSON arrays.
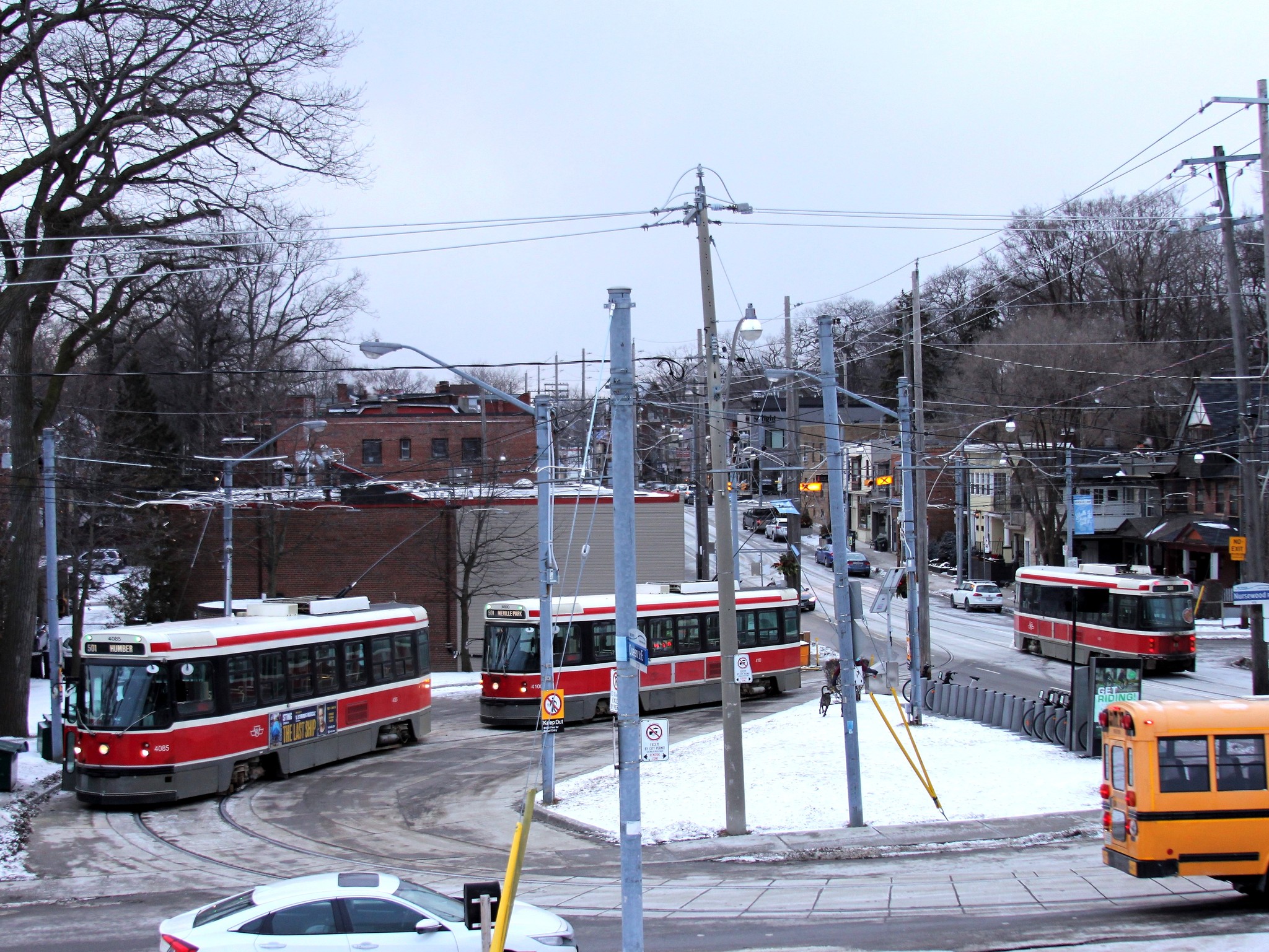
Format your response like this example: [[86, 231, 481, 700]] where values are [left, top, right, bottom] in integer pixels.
[[75, 548, 127, 574], [950, 579, 1003, 613]]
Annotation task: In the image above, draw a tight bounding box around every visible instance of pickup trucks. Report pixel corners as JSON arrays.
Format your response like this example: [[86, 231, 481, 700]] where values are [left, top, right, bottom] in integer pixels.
[[742, 507, 782, 533]]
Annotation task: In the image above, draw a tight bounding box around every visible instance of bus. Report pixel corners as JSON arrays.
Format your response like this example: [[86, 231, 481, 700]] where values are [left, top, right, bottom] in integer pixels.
[[1099, 696, 1269, 909], [1014, 497, 1196, 677], [37, 504, 455, 808], [464, 501, 802, 729]]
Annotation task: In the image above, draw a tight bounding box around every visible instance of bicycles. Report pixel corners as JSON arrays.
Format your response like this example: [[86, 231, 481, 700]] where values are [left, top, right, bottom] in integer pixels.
[[902, 661, 980, 711], [1020, 688, 1089, 751]]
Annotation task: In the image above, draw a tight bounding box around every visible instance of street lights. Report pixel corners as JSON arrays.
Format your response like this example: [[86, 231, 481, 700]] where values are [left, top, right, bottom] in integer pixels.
[[1194, 446, 1249, 629], [222, 419, 328, 616], [998, 453, 1073, 560], [707, 304, 764, 837], [919, 418, 1016, 681], [765, 369, 923, 726], [358, 337, 556, 804]]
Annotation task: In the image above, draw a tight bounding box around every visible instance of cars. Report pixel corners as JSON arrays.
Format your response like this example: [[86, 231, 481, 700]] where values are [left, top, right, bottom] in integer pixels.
[[815, 544, 871, 577], [156, 869, 579, 952], [642, 481, 713, 506], [766, 580, 815, 612], [764, 518, 788, 543]]
